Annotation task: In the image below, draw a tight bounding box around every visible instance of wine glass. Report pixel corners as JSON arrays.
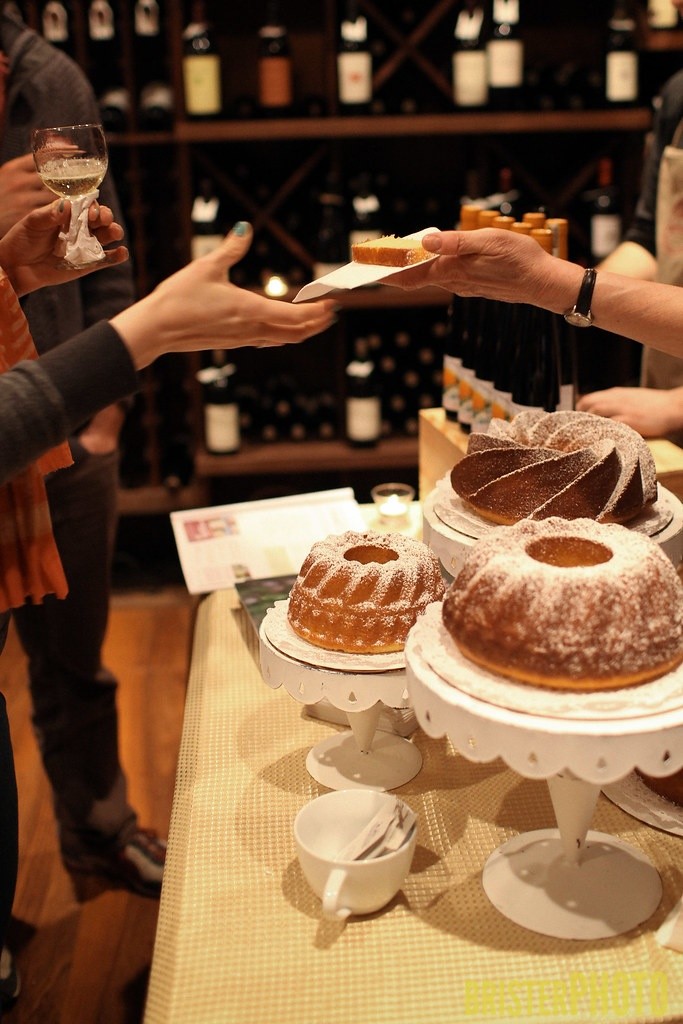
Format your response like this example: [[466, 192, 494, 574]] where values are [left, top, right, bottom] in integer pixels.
[[31, 123, 108, 271]]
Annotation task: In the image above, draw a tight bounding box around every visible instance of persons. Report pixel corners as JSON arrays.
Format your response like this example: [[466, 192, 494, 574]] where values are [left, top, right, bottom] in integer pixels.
[[575, 0, 683, 439], [0, 0, 343, 1015], [373, 228, 683, 361]]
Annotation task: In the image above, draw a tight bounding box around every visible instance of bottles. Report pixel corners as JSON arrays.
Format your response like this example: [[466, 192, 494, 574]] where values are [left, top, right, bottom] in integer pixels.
[[585, 149, 622, 268], [384, 321, 444, 435], [41, 1, 175, 136], [441, 203, 575, 435], [257, 0, 294, 118], [313, 182, 381, 282], [191, 175, 231, 285], [602, 0, 641, 110], [336, 0, 372, 119], [342, 336, 384, 450], [183, 0, 222, 120], [448, 0, 490, 113], [202, 347, 240, 454], [237, 366, 340, 444], [485, 0, 526, 113]]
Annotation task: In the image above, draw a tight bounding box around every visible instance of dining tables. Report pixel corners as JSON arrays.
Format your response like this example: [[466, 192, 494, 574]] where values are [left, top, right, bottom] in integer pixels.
[[141, 499, 682, 1023]]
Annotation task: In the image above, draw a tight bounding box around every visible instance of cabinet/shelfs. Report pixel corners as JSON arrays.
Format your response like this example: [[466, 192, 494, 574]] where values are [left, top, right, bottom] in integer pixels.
[[84, 102, 657, 516]]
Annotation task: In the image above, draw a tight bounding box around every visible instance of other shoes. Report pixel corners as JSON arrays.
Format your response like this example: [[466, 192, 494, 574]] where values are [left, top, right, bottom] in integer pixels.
[[0, 946, 20, 1002]]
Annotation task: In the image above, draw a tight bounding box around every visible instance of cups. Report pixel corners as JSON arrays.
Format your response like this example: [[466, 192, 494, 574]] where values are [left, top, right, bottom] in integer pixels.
[[292, 790, 417, 922]]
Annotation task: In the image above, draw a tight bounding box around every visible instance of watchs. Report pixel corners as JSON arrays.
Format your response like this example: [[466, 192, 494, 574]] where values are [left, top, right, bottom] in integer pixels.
[[563, 269, 598, 328]]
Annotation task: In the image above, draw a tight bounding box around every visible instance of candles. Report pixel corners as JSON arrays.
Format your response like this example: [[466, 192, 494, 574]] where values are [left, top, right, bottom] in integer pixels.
[[381, 490, 409, 524]]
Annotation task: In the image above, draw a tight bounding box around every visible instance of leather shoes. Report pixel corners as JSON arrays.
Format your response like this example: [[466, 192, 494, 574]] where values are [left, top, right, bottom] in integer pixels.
[[67, 831, 167, 903]]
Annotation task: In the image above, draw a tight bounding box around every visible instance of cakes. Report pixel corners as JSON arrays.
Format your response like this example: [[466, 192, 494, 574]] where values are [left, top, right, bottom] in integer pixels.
[[450, 409, 659, 530], [350, 234, 440, 268], [286, 531, 447, 654], [441, 516, 683, 693]]
